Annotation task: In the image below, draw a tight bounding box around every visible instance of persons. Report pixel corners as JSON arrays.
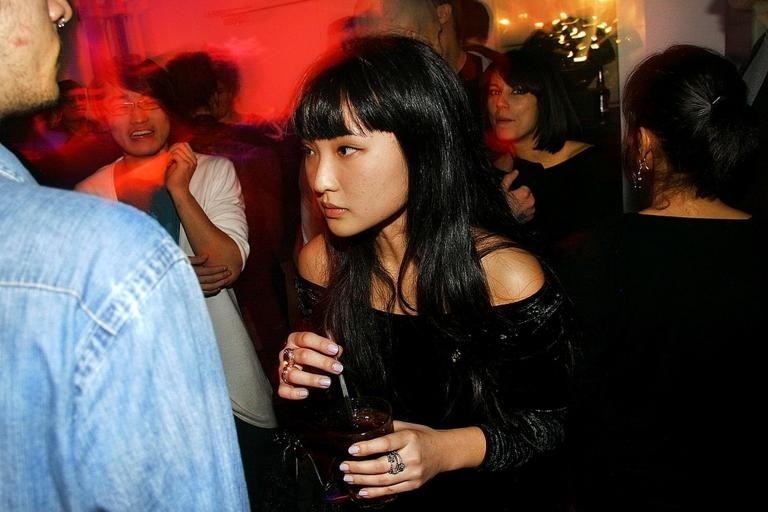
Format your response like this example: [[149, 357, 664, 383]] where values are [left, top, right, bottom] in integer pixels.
[[1, 0, 503, 511], [566, 44, 768, 511], [480, 49, 612, 282], [260, 33, 583, 512]]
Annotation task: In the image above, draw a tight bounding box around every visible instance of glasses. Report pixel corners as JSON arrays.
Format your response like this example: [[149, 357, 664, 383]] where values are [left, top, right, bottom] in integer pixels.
[[110, 99, 162, 115]]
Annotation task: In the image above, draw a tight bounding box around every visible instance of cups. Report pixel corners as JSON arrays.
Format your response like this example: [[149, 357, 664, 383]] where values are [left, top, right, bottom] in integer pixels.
[[321, 396, 402, 510]]
